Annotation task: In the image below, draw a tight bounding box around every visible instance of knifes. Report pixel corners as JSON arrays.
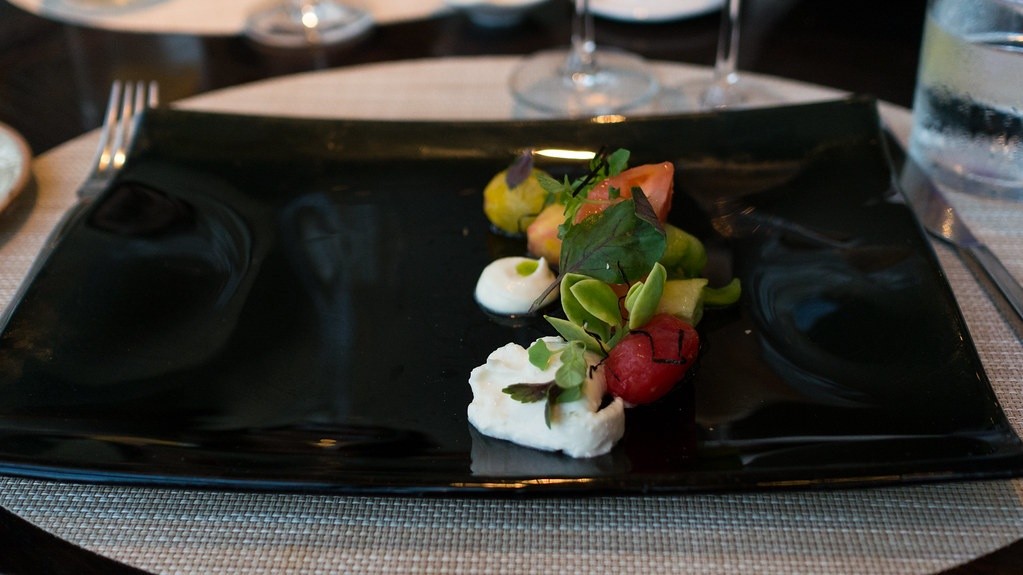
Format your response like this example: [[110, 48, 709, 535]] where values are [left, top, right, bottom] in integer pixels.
[[884, 127, 1023, 322]]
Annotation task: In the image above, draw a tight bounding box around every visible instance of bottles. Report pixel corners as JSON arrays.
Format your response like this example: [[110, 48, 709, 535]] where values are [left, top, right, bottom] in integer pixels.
[[906, 0, 1023, 205]]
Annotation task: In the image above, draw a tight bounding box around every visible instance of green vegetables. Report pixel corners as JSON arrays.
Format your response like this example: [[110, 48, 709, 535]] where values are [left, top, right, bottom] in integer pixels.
[[504, 145, 742, 429]]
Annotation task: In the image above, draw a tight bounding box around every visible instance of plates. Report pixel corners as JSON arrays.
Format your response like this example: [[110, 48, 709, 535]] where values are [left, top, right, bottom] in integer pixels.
[[0, 98, 1022, 497]]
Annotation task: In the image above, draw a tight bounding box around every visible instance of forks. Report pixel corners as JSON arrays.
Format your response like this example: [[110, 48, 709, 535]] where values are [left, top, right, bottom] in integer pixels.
[[2, 80, 159, 333]]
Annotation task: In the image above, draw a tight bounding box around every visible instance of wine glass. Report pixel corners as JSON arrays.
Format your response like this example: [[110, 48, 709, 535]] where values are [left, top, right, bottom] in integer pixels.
[[658, 1, 785, 115], [248, 1, 375, 47], [509, 0, 658, 115]]
